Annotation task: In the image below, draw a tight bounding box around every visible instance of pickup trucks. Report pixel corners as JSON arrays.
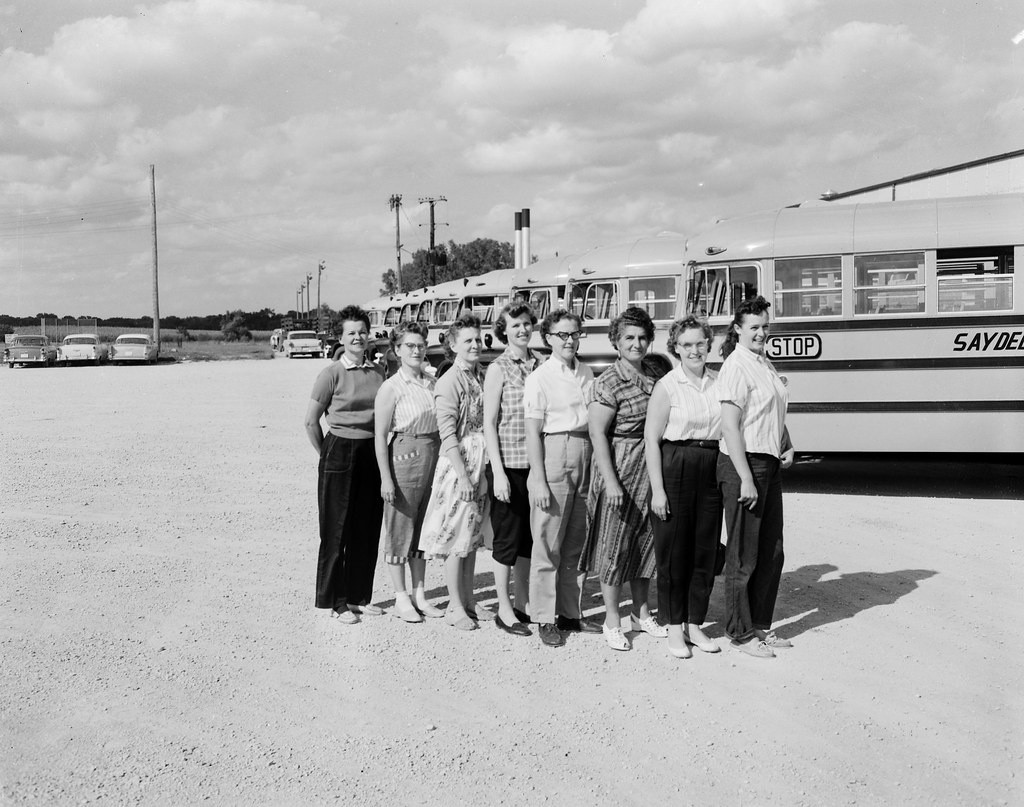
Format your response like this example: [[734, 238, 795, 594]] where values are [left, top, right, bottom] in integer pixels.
[[3, 334, 57, 368]]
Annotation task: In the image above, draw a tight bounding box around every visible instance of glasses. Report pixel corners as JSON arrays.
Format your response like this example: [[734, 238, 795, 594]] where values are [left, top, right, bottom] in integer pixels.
[[678, 337, 711, 350], [546, 330, 582, 341]]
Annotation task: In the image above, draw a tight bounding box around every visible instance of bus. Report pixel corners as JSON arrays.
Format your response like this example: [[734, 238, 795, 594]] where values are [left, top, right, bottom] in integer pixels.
[[327, 228, 686, 380], [671, 191, 1023, 463]]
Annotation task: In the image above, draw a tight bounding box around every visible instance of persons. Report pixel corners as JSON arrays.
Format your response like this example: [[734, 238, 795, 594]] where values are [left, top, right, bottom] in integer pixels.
[[643, 316, 724, 658], [715, 294, 794, 658], [585, 306, 668, 651], [419, 315, 497, 631], [522, 310, 603, 646], [374, 321, 445, 622], [304, 304, 388, 624], [482, 303, 549, 637]]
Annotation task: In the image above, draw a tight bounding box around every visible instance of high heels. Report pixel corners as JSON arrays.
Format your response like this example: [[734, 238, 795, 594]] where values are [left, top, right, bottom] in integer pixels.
[[602, 621, 631, 651], [630, 609, 666, 637]]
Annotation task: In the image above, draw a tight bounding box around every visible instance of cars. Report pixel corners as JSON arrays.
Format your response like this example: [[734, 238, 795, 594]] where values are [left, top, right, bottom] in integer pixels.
[[269, 328, 288, 352], [282, 330, 324, 360], [107, 334, 159, 365], [56, 333, 108, 367]]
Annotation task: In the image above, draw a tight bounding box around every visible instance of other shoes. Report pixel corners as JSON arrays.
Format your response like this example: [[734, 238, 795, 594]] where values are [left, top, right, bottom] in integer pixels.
[[331, 606, 360, 624], [667, 634, 692, 658], [346, 601, 383, 616], [444, 606, 475, 631], [683, 628, 721, 653], [465, 602, 497, 620], [755, 629, 791, 649], [393, 603, 422, 621], [411, 595, 444, 618], [725, 635, 774, 658]]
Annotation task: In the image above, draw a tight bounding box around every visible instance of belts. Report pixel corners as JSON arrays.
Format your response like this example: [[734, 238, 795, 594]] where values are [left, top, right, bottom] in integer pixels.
[[546, 430, 590, 438]]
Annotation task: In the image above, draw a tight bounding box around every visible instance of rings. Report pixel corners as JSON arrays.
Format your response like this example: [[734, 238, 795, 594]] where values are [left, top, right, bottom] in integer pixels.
[[496, 495, 499, 498]]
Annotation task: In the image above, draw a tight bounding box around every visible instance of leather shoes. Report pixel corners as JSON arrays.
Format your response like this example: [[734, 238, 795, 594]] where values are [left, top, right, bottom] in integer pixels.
[[539, 623, 563, 647], [495, 614, 531, 636], [513, 607, 531, 623], [558, 613, 603, 633]]
